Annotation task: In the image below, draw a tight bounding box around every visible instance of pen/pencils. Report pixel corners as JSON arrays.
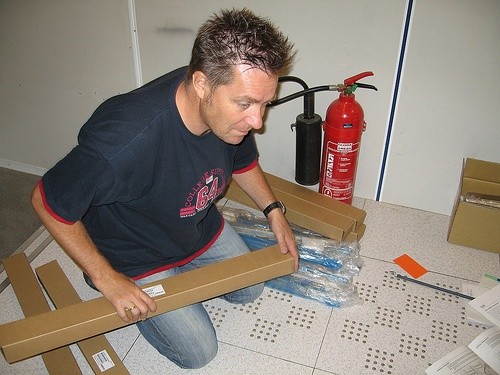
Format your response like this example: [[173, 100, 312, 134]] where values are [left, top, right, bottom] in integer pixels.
[[487, 273, 500, 282]]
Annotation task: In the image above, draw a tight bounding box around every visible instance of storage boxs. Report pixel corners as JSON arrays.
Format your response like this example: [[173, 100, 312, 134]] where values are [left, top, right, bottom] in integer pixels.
[[447, 157, 500, 256], [0, 227, 296, 375], [222, 169, 367, 243]]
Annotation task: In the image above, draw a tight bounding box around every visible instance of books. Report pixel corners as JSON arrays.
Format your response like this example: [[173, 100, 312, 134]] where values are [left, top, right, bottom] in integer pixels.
[[465, 273, 500, 327], [425, 325, 500, 375]]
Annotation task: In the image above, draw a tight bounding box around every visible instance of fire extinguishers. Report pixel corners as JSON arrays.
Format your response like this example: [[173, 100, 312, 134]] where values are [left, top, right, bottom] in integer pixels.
[[266, 72, 378, 206]]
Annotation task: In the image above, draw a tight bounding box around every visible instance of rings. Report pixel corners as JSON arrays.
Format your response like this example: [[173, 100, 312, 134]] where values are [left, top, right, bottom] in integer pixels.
[[128, 306, 135, 311]]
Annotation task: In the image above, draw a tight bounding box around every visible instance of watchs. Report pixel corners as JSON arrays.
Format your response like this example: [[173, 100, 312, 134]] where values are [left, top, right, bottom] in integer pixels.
[[263, 200, 286, 220]]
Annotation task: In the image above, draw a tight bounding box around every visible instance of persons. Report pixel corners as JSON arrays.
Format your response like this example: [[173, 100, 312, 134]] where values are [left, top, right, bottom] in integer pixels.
[[31, 6, 299, 369]]
